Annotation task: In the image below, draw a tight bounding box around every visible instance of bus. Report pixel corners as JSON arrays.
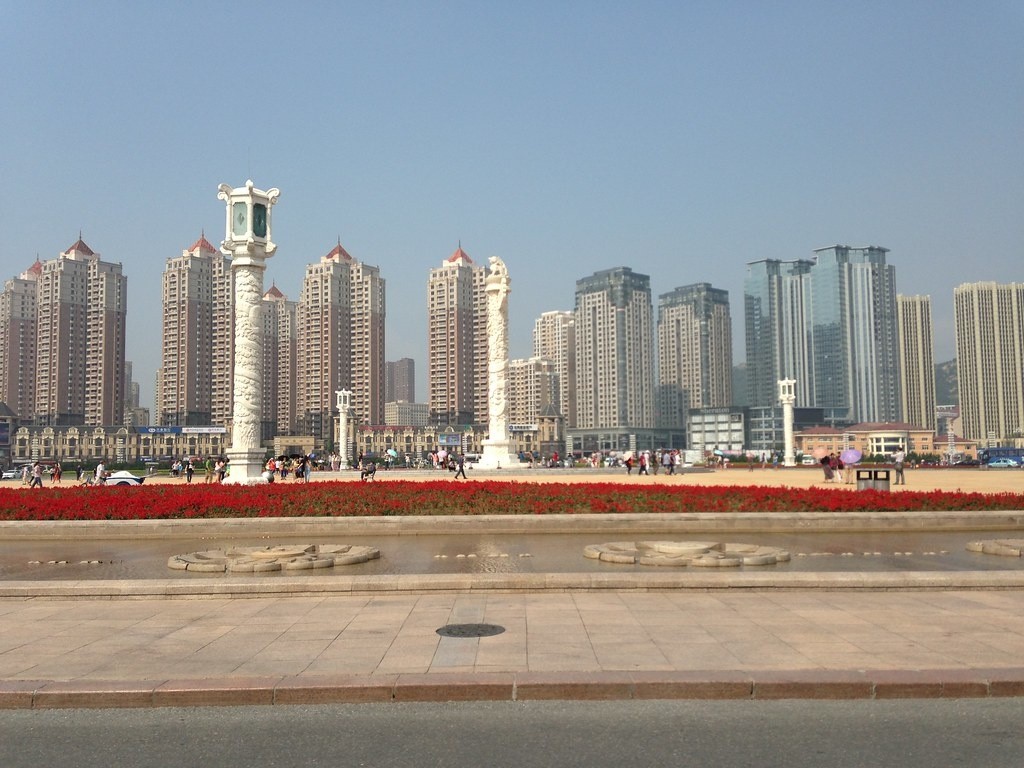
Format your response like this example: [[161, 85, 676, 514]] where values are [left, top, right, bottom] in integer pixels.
[[982, 447, 1024, 464]]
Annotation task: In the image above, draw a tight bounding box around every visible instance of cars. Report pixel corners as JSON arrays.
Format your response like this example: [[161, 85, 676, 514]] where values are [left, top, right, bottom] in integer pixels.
[[988, 459, 1019, 468], [2, 469, 22, 480], [953, 459, 980, 467], [802, 455, 818, 465], [924, 459, 936, 466], [40, 465, 52, 475]]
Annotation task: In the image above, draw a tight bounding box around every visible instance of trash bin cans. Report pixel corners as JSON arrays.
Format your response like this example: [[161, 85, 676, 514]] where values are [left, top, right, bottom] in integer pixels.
[[856, 469, 890, 492]]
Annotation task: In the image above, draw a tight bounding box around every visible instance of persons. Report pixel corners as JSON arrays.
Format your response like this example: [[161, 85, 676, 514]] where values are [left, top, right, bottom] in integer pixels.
[[822, 452, 854, 484], [715, 455, 723, 468], [21, 457, 230, 487], [530, 448, 538, 468], [358, 451, 363, 470], [772, 454, 778, 471], [625, 448, 684, 475], [454, 454, 468, 479], [426, 449, 456, 471], [565, 453, 573, 467], [748, 457, 754, 472], [268, 450, 341, 484], [361, 461, 376, 480], [385, 450, 395, 471], [518, 451, 525, 461], [760, 452, 767, 469], [591, 449, 601, 468], [405, 454, 413, 469], [891, 447, 905, 485], [553, 452, 559, 467]]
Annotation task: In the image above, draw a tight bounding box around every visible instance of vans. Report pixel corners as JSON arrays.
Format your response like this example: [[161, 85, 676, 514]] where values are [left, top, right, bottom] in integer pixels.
[[464, 453, 484, 463]]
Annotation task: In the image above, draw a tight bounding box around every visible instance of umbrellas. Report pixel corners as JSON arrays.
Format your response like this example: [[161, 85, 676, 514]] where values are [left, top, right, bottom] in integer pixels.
[[624, 451, 633, 461], [388, 449, 397, 458], [840, 449, 861, 463], [290, 453, 300, 459], [746, 453, 755, 458], [714, 450, 723, 455], [278, 455, 290, 461]]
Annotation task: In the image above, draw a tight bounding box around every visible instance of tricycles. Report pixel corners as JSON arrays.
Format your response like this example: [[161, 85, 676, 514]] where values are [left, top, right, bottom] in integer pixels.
[[77, 471, 147, 487]]
